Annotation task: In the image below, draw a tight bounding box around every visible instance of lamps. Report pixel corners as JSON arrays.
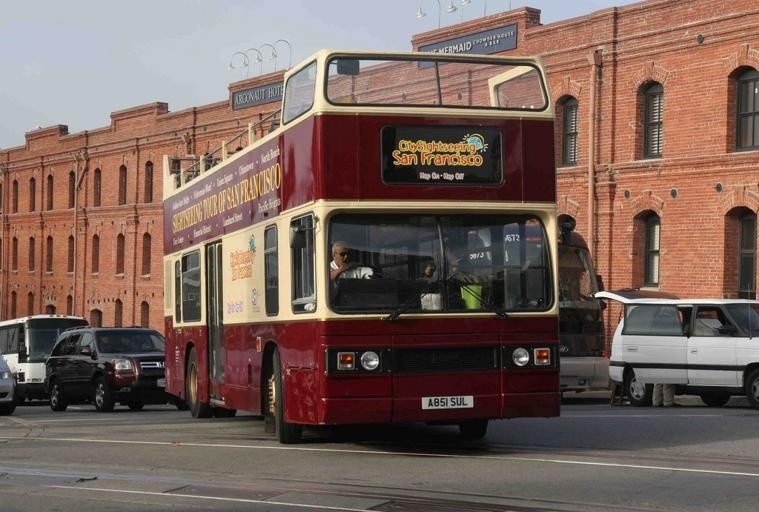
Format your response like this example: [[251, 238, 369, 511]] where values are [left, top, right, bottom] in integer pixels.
[[230, 39, 292, 80], [462, 0, 486, 17], [417, 0, 441, 31], [448, 0, 462, 24]]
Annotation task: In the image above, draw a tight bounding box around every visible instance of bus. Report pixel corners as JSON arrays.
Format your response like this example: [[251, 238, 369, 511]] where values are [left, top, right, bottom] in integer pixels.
[[161, 48, 562, 443], [465, 214, 613, 403], [0, 313, 92, 406]]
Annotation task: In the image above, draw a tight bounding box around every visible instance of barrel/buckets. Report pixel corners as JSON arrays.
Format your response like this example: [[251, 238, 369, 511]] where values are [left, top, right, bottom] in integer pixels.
[[461, 285, 482, 309]]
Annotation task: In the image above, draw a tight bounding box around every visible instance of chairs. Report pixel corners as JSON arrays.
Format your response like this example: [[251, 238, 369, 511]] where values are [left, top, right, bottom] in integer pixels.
[[694, 317, 724, 337]]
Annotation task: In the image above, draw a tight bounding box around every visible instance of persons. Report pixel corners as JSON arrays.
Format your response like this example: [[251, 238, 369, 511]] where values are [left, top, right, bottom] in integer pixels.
[[649, 306, 682, 407], [420, 264, 436, 279], [444, 238, 475, 282], [329, 240, 375, 282]]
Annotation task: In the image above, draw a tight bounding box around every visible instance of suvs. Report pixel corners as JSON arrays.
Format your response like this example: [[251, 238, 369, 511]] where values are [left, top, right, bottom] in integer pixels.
[[587, 287, 759, 411], [44, 326, 189, 413]]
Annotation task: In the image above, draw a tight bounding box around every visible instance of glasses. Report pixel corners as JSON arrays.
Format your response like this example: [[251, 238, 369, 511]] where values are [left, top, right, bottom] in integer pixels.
[[334, 251, 348, 256]]
